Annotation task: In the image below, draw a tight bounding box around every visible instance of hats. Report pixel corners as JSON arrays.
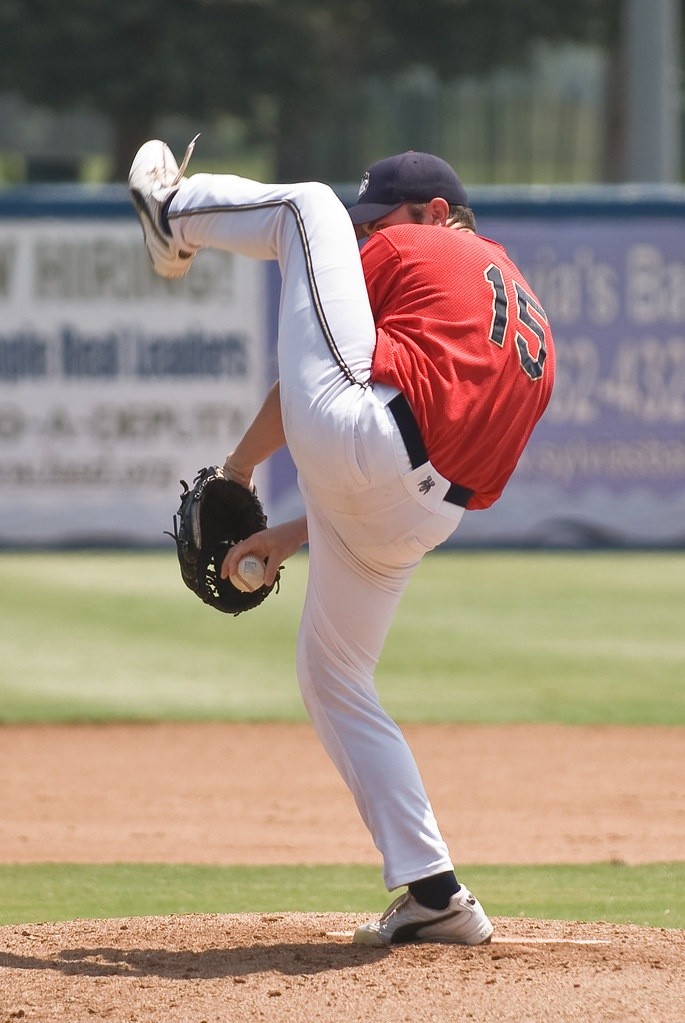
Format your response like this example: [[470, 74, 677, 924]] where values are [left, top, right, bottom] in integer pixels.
[[347, 150, 468, 241]]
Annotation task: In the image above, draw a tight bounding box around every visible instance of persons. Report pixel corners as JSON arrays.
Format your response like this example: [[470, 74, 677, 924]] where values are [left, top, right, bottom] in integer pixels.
[[129, 134, 556, 946]]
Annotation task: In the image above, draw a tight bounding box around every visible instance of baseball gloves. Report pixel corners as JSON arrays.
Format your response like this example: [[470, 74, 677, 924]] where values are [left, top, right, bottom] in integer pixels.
[[162, 463, 287, 618]]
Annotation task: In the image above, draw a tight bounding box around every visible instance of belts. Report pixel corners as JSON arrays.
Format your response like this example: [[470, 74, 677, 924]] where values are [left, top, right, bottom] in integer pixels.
[[387, 394, 473, 507]]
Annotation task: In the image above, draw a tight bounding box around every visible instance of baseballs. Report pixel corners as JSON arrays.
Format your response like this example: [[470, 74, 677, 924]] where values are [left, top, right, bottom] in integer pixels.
[[228, 553, 267, 593]]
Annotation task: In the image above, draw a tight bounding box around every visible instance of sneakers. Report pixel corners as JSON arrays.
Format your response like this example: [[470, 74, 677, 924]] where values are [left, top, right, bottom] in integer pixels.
[[128, 132, 200, 278], [353, 884, 494, 945]]
